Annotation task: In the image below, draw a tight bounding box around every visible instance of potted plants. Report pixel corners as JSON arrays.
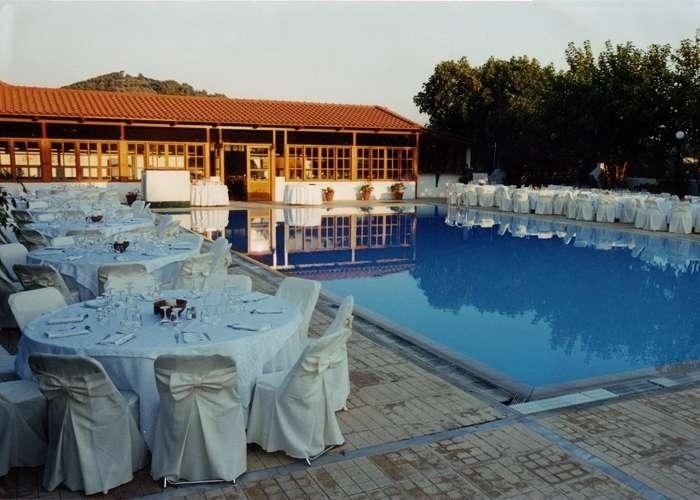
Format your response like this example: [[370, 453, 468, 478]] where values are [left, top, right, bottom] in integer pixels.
[[391, 183, 408, 200], [360, 184, 375, 200]]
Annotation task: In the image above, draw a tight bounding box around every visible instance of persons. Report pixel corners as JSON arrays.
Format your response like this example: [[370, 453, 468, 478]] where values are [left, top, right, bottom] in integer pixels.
[[464, 164, 474, 184]]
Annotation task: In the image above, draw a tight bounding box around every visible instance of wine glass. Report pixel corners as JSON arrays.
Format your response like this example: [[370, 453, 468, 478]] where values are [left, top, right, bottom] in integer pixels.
[[66, 274, 286, 344], [19, 178, 193, 268]]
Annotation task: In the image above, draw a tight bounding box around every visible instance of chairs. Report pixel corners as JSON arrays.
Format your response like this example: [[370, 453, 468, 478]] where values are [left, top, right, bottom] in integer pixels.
[[444, 183, 700, 234]]
